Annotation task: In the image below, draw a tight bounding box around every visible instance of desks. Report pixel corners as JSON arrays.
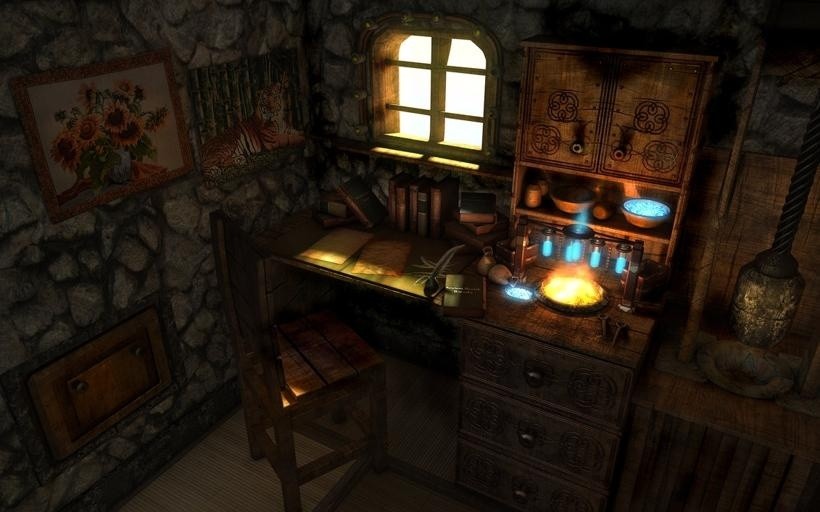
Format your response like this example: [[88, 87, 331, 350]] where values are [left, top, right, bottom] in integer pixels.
[[240, 202, 655, 512]]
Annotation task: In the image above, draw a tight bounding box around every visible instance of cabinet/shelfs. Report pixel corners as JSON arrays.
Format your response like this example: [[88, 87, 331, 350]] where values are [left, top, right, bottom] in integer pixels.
[[512, 39, 722, 283]]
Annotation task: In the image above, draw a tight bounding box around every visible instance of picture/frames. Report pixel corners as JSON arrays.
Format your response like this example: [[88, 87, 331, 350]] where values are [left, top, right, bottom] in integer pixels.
[[9, 46, 196, 225]]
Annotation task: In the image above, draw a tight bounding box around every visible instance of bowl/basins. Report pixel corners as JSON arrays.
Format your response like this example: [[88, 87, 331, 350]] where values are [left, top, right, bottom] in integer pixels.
[[621, 198, 673, 228], [550, 189, 598, 214]]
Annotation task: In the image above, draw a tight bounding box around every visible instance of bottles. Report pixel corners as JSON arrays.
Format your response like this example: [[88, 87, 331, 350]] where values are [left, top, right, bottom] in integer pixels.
[[538, 224, 633, 278], [525, 180, 548, 208]]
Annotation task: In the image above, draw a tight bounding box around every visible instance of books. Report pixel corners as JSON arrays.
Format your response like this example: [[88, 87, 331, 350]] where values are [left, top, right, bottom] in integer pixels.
[[320, 171, 509, 255], [444, 271, 488, 312]]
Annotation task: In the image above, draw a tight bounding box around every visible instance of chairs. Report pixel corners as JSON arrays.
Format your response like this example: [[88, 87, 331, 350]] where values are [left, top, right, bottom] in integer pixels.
[[196, 212, 392, 512]]
